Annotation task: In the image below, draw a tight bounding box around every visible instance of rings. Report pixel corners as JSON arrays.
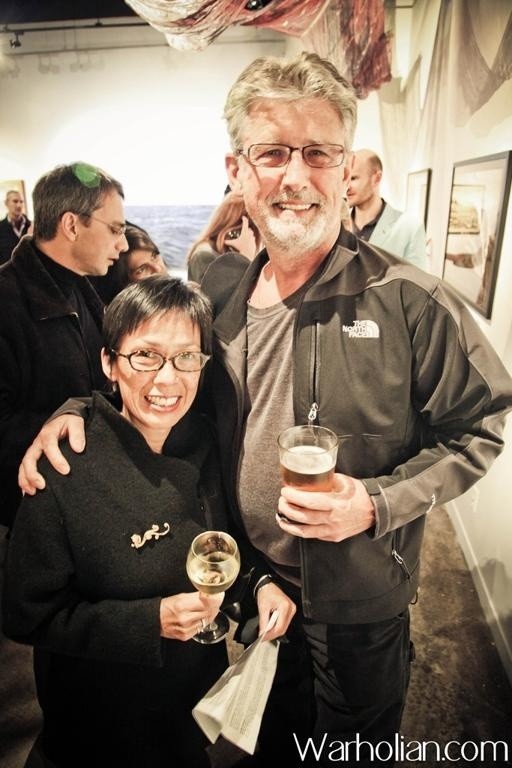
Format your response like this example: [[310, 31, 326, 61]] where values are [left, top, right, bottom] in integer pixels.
[[197, 627, 202, 637], [200, 618, 208, 630]]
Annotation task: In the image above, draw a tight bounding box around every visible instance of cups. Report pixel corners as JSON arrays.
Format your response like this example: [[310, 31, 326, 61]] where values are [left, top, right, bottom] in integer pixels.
[[276, 424, 340, 494]]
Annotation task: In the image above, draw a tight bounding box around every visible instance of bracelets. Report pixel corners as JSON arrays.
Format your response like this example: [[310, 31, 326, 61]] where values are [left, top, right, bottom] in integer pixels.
[[253, 572, 273, 595]]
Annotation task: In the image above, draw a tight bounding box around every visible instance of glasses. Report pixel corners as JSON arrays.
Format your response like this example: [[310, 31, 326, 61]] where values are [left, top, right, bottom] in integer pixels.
[[81, 213, 126, 236], [236, 143, 344, 167], [111, 349, 212, 372]]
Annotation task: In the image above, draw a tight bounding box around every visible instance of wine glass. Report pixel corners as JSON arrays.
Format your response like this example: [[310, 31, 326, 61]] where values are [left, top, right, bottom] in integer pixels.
[[186, 530, 243, 646]]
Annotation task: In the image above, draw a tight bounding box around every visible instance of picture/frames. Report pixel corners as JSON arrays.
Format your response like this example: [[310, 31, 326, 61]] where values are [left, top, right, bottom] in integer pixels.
[[406, 169, 432, 232], [442, 149, 512, 320]]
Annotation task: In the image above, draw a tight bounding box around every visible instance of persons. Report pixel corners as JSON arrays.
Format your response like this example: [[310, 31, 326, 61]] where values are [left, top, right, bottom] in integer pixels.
[[105, 225, 168, 299], [1, 162, 130, 493], [187, 192, 261, 287], [345, 149, 429, 274], [0, 190, 33, 262], [0, 275, 299, 762], [15, 49, 511, 767]]
